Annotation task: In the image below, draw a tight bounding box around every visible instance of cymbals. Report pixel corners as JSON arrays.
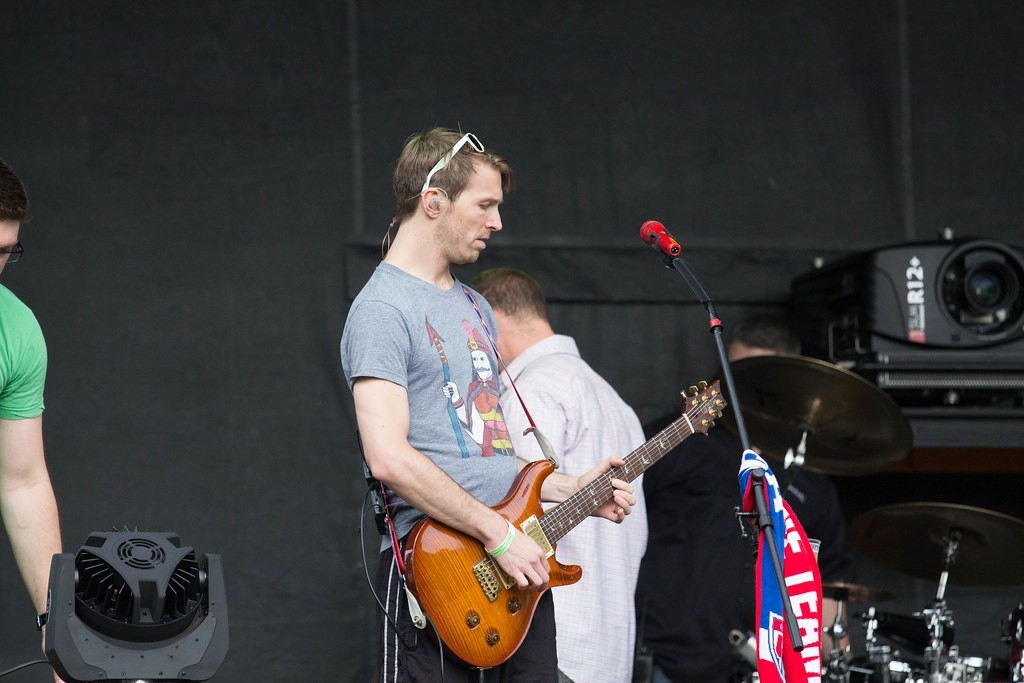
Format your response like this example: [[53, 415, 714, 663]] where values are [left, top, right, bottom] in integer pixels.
[[714, 351, 915, 479], [842, 500, 1024, 592]]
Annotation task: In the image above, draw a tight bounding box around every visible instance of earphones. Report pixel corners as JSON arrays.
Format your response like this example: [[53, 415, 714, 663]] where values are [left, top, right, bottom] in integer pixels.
[[431, 198, 441, 210]]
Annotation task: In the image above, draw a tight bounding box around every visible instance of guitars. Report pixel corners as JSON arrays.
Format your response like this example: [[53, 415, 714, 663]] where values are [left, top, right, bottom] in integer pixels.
[[396, 377, 730, 671]]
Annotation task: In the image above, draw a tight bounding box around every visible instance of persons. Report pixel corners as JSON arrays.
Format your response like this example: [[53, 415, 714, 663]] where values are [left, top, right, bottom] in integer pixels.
[[470, 265, 648, 683], [336, 127, 637, 682], [634, 315, 844, 683], [0, 162, 70, 683]]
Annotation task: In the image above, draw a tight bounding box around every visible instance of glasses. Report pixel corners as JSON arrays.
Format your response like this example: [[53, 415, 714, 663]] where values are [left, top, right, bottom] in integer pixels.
[[420, 133, 485, 197], [0, 242, 24, 264]]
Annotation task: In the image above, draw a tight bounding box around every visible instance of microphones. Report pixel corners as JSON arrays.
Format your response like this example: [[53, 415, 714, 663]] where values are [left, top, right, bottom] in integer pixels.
[[639, 220, 682, 256]]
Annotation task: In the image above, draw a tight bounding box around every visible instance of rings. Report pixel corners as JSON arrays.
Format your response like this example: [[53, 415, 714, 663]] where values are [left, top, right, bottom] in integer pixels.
[[615, 506, 621, 513]]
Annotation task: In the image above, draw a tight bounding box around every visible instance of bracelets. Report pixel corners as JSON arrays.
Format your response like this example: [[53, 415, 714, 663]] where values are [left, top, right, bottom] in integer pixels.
[[36, 613, 48, 631], [483, 518, 516, 559]]
